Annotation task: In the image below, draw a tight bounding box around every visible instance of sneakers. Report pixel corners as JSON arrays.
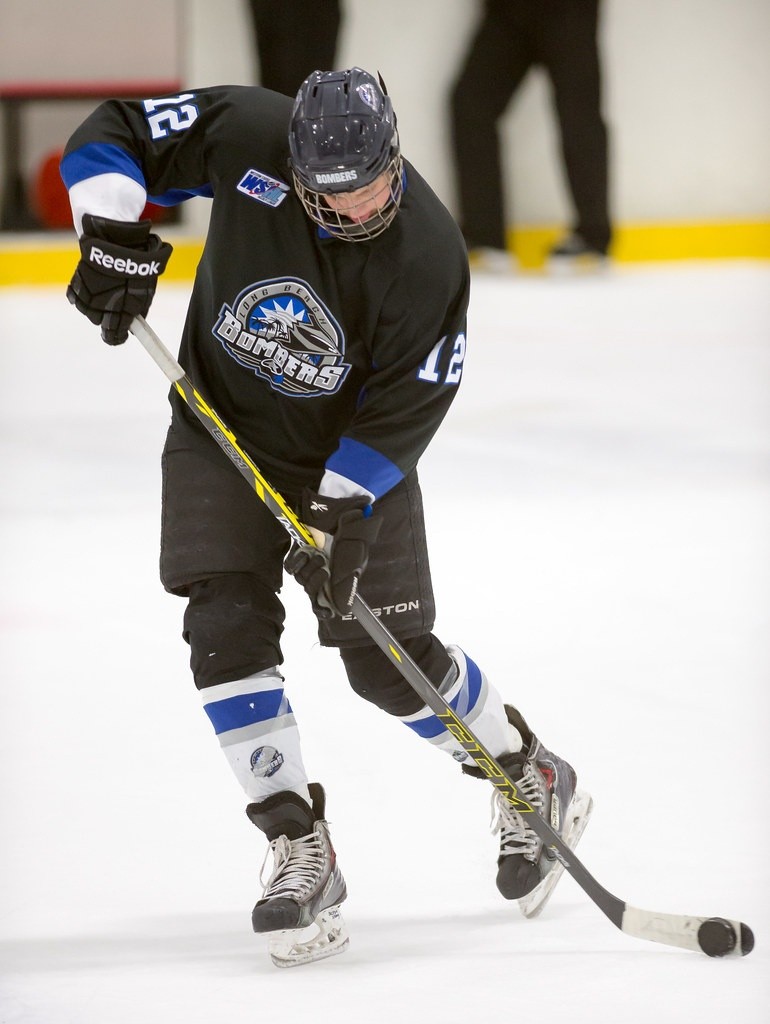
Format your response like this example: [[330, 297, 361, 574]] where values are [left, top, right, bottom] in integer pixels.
[[460, 706, 592, 918], [245, 781, 349, 966]]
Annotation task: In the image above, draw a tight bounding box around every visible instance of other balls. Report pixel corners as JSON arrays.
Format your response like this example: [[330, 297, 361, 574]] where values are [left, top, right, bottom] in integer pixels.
[[697, 917, 736, 958]]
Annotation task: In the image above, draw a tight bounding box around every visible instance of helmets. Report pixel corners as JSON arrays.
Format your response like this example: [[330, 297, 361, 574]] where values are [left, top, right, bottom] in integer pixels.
[[288, 65, 397, 194]]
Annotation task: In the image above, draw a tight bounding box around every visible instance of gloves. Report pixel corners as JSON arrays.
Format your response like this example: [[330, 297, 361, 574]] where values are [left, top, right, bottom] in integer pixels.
[[284, 488, 385, 619], [65, 210, 173, 345]]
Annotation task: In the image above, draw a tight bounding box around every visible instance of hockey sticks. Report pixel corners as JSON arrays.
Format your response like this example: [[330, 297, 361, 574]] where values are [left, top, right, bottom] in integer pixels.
[[130, 310, 756, 957]]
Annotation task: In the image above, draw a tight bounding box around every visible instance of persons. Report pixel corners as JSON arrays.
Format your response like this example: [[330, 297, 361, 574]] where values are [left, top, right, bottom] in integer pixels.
[[252, 0, 611, 258], [60, 68, 593, 966]]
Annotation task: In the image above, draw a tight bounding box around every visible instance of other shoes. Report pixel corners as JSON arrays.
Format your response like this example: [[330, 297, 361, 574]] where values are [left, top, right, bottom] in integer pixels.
[[453, 221, 506, 253], [546, 230, 606, 260]]
